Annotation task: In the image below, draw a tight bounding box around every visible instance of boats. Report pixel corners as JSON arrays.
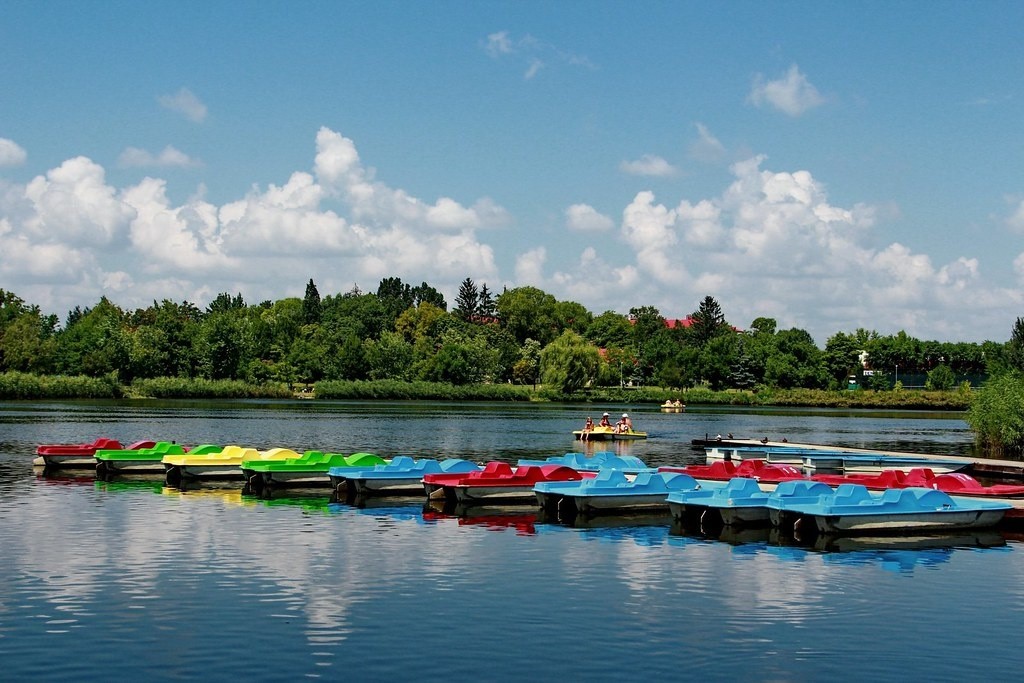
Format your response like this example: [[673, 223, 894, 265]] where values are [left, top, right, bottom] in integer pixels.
[[29, 437, 1023, 549], [572, 424, 648, 441], [660, 400, 686, 414]]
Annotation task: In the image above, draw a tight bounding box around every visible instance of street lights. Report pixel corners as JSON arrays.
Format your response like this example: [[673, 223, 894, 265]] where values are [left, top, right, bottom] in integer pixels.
[[621, 362, 623, 387], [896, 365, 899, 384]]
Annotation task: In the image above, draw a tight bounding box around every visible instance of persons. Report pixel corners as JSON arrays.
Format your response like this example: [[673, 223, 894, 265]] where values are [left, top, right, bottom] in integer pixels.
[[580, 412, 632, 440], [666, 399, 680, 405]]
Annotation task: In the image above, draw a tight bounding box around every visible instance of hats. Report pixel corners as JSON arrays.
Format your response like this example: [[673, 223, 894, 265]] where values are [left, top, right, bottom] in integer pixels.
[[603, 413, 610, 416], [622, 413, 628, 418]]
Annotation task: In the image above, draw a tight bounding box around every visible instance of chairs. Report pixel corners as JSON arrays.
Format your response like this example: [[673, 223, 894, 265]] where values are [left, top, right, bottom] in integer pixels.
[[39, 427, 1024, 515]]
